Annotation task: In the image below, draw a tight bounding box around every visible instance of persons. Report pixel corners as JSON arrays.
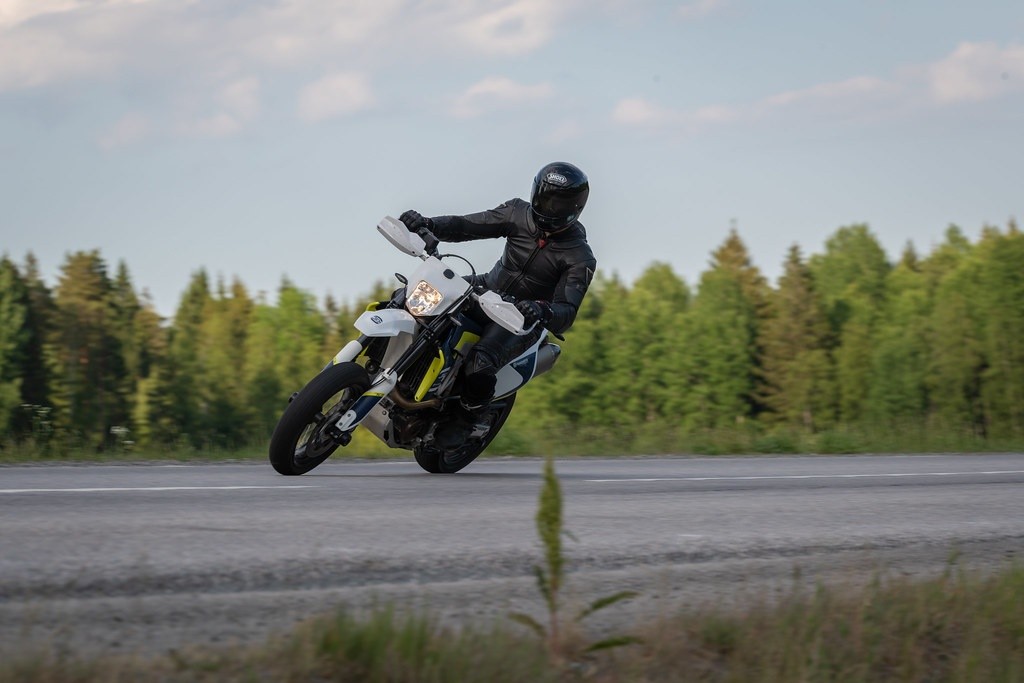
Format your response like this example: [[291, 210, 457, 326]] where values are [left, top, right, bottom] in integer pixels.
[[399, 161, 597, 454]]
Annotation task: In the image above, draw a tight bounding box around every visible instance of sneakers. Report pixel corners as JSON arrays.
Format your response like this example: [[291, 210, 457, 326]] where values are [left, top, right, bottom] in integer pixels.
[[433, 406, 480, 451]]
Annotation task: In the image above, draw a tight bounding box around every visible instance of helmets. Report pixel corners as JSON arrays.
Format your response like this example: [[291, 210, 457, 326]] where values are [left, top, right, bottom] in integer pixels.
[[530, 162, 589, 233]]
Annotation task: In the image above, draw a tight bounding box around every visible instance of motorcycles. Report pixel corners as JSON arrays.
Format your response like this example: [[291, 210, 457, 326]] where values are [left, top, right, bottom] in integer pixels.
[[269, 213, 565, 479]]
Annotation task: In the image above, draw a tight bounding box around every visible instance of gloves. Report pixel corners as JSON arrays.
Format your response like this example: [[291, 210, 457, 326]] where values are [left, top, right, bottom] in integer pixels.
[[399, 210, 434, 232], [515, 299, 546, 328]]
